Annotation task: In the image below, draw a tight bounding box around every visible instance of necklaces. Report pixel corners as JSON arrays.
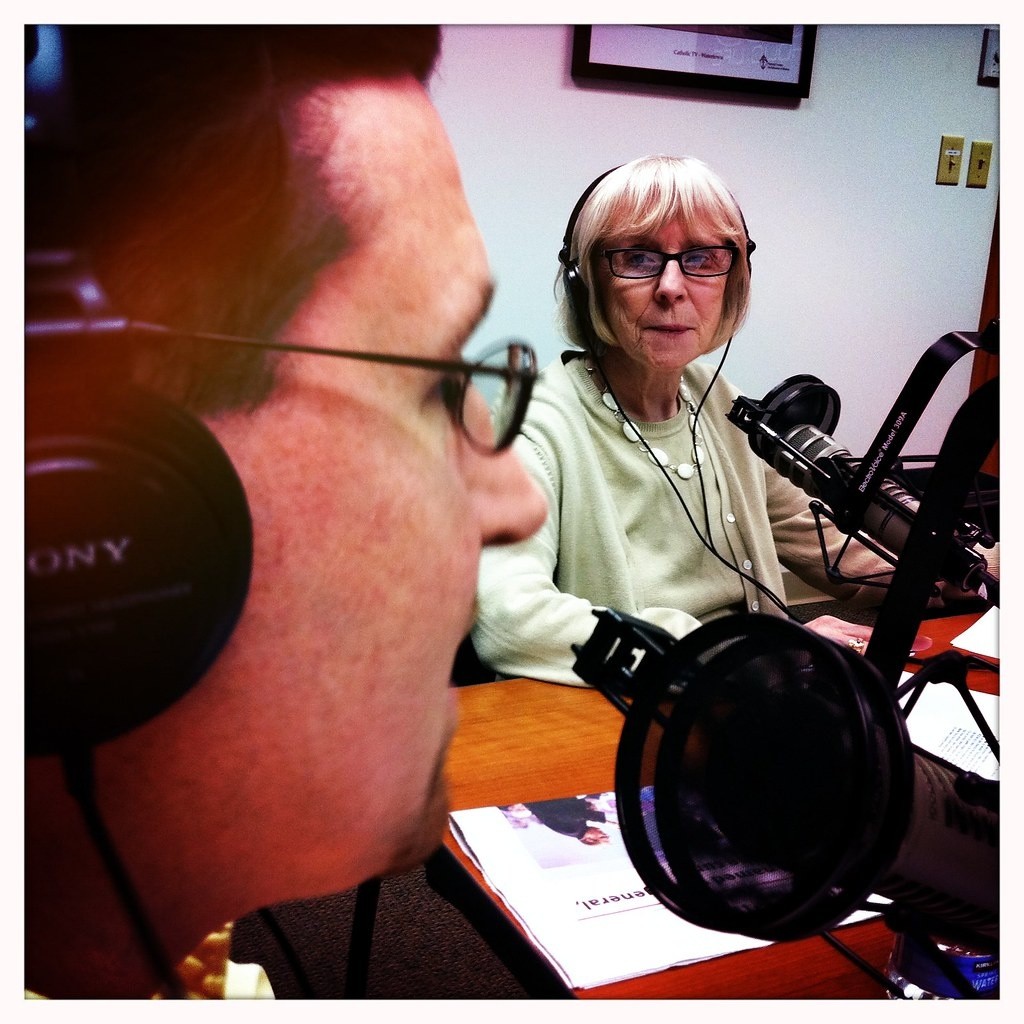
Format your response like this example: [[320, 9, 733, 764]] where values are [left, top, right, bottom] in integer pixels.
[[586, 353, 706, 480]]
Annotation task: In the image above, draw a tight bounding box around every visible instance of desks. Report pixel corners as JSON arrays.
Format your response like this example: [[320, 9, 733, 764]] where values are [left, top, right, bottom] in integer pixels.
[[345, 614, 1000, 999]]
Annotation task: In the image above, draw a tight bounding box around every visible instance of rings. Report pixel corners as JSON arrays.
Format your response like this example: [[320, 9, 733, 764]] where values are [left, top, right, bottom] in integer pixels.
[[847, 637, 864, 653]]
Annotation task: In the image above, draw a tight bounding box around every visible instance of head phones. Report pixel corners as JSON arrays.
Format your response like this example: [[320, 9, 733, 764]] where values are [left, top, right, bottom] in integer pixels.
[[23, 173, 263, 763], [558, 165, 757, 355]]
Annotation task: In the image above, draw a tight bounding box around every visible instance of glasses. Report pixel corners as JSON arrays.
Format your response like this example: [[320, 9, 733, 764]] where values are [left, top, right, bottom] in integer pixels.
[[601, 246, 739, 279], [24, 320, 539, 456]]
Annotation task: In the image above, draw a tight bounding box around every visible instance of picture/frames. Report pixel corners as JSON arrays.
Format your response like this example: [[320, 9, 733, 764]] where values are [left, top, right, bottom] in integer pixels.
[[572, 24, 817, 98], [977, 29, 1000, 89]]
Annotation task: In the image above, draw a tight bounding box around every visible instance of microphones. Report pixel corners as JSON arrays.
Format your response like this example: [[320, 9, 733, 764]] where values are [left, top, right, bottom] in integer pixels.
[[771, 424, 986, 592], [885, 752, 999, 919]]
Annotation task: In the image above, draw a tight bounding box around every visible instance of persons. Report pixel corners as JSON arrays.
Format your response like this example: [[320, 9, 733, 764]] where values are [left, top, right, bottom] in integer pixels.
[[461, 157, 1000, 688], [27, 25, 547, 998], [518, 794, 608, 846]]
